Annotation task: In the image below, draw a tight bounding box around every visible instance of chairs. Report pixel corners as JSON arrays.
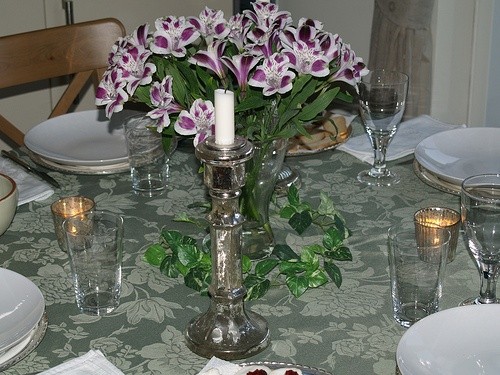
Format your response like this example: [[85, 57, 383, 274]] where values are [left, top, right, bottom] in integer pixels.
[[0, 18, 127, 151]]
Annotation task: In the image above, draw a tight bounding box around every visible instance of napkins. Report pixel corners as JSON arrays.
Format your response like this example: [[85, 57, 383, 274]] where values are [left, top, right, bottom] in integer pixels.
[[34, 350, 125, 375], [0, 157, 54, 206], [336, 112, 453, 167], [197, 356, 241, 375]]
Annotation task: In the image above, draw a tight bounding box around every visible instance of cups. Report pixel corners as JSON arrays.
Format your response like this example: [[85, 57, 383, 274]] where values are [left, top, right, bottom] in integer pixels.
[[123, 114, 179, 198], [62, 210, 123, 316], [387, 221, 451, 328]]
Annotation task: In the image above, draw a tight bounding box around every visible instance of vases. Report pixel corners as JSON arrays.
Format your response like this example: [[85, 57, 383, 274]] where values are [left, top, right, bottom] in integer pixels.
[[241, 139, 288, 254]]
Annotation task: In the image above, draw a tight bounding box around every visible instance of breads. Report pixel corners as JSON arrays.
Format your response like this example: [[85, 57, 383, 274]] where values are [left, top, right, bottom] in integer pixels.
[[296, 117, 348, 150]]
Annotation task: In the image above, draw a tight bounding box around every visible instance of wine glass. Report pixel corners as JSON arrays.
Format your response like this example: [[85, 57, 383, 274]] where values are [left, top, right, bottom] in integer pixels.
[[458, 174, 500, 306], [357, 69, 409, 187]]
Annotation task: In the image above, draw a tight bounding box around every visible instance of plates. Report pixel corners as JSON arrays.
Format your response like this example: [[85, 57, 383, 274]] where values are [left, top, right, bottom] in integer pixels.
[[24, 109, 160, 166], [0, 267, 45, 352], [414, 127, 500, 186], [396, 304, 500, 375]]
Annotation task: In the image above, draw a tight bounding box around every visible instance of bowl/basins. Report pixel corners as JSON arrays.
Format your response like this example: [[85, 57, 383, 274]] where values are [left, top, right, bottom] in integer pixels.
[[0, 173, 17, 235]]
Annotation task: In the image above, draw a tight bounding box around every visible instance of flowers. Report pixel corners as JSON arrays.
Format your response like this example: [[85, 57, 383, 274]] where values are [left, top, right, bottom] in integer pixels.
[[93, 1, 370, 245]]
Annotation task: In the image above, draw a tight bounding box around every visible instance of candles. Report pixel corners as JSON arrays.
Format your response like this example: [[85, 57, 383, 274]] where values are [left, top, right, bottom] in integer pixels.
[[215, 88, 235, 147]]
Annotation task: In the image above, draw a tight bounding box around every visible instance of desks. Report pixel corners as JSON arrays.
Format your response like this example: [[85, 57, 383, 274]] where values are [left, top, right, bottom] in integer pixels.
[[0, 123, 500, 375]]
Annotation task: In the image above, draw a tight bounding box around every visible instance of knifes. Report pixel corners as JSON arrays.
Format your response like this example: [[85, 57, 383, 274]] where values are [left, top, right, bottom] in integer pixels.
[[1, 149, 60, 189]]
